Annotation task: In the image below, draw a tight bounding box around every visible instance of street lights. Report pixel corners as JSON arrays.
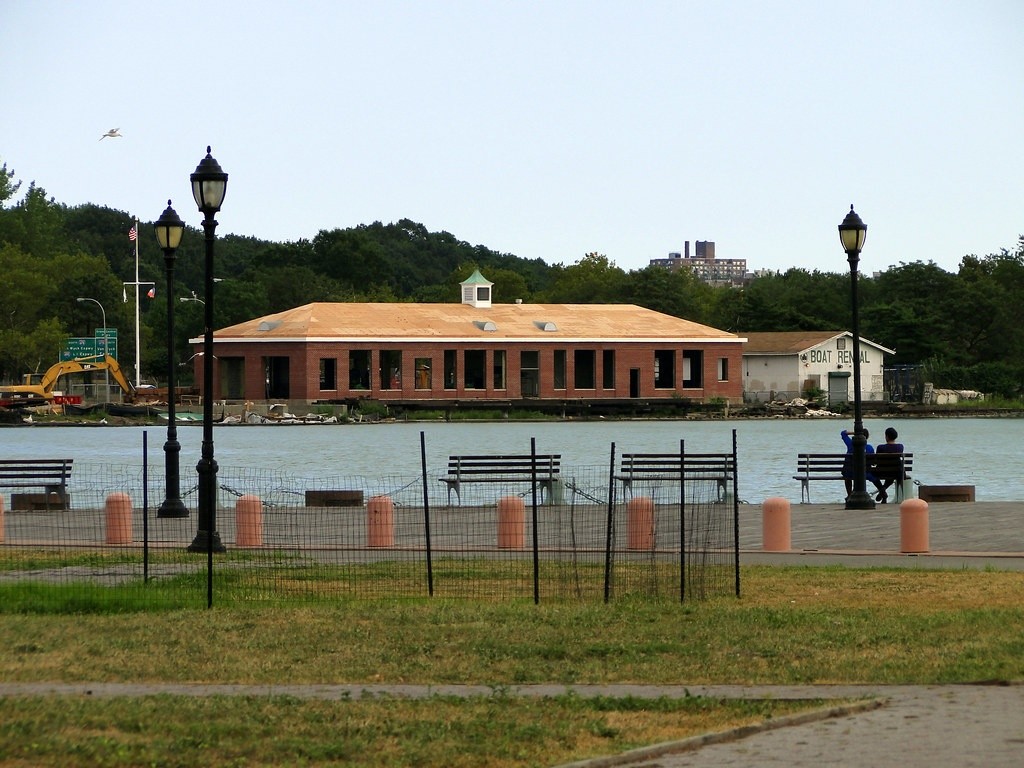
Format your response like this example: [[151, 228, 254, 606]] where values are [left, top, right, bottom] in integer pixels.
[[838, 203, 877, 511], [151, 199, 184, 519], [77, 298, 110, 403], [185, 144, 228, 554]]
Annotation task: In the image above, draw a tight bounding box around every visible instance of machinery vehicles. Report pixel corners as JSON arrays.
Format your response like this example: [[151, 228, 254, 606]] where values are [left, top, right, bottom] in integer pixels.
[[0, 350, 147, 418]]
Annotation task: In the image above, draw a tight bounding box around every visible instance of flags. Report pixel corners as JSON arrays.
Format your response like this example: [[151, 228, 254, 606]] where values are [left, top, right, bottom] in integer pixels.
[[129, 225, 136, 241]]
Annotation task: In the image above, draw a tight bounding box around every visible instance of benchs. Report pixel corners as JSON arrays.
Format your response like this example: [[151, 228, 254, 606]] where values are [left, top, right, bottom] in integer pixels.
[[793, 453, 914, 504], [0, 459, 74, 510], [613, 454, 735, 503], [439, 455, 560, 507]]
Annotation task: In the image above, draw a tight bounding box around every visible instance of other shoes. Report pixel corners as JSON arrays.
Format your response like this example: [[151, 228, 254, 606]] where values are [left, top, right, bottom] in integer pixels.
[[882, 493, 887, 503], [876, 493, 882, 501]]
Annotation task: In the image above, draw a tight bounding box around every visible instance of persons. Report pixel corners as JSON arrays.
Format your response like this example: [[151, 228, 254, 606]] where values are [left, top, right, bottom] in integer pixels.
[[873, 427, 904, 504], [841, 429, 874, 501]]
